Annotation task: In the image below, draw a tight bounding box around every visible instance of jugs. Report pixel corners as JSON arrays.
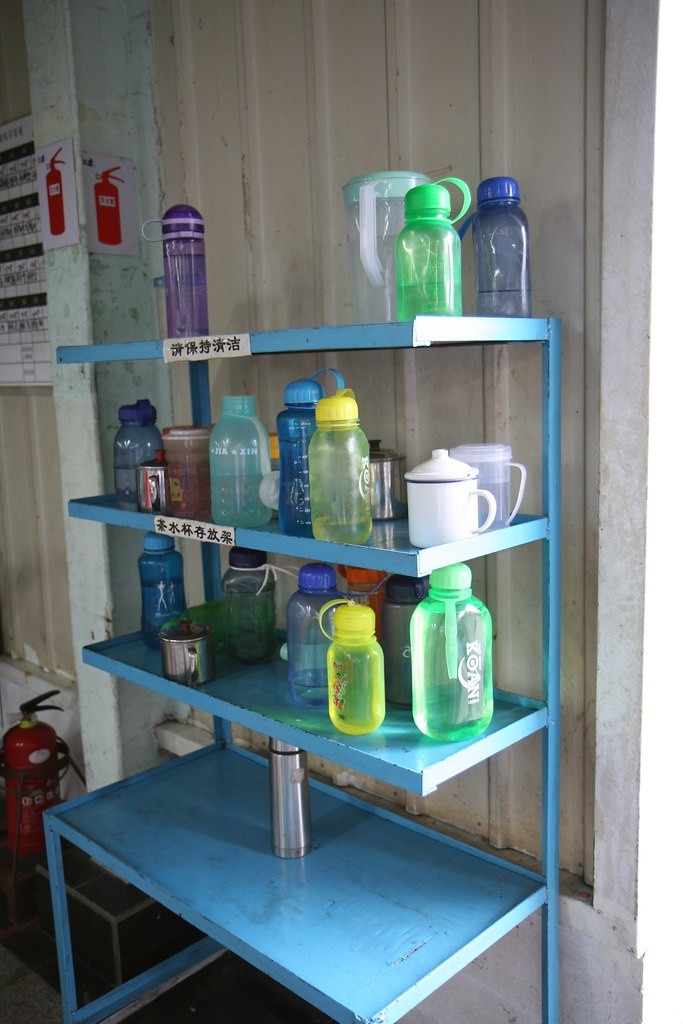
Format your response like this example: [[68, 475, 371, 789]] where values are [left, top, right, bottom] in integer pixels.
[[342, 171, 433, 325]]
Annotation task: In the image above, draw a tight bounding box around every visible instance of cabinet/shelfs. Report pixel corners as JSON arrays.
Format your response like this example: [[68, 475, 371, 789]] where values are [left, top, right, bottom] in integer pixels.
[[41, 316, 559, 1024]]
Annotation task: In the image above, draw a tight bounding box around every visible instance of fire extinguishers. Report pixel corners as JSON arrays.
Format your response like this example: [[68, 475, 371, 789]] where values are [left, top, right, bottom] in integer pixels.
[[2, 689, 64, 859]]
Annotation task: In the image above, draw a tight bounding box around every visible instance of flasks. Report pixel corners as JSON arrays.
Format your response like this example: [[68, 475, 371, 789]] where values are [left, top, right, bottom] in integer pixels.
[[270, 738, 305, 859]]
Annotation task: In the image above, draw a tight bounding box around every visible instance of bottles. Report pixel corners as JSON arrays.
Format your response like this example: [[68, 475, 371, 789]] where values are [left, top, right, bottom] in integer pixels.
[[114, 367, 495, 738], [396, 177, 471, 321], [142, 204, 210, 337], [457, 175, 531, 318]]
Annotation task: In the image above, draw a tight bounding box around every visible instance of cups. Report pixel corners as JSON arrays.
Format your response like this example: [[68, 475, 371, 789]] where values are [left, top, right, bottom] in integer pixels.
[[370, 440, 407, 522], [134, 452, 172, 515], [159, 612, 217, 686], [403, 442, 528, 548]]
